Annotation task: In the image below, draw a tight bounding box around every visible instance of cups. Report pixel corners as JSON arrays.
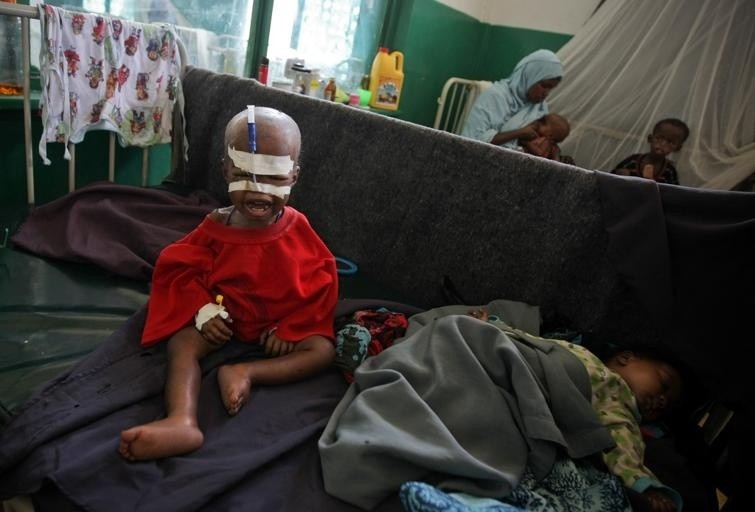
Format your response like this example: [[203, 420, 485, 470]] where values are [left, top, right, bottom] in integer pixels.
[[357, 88, 373, 107]]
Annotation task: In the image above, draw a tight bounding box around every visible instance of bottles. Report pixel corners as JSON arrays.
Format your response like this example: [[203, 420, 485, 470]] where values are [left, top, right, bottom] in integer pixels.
[[323, 77, 336, 101], [258, 63, 268, 83]]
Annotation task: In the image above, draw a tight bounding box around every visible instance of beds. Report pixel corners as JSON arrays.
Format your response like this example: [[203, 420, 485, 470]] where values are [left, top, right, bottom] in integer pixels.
[[1, 1, 188, 203], [435, 75, 755, 191]]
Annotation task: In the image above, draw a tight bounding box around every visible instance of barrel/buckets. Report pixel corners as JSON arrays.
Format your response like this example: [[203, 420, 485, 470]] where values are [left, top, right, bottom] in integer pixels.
[[367, 47, 405, 110]]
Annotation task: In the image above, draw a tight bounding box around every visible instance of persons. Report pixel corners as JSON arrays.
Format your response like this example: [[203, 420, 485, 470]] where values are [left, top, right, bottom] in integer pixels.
[[393, 304, 692, 510], [610, 117, 691, 186], [460, 48, 578, 167], [519, 113, 571, 160], [116, 104, 340, 462]]
